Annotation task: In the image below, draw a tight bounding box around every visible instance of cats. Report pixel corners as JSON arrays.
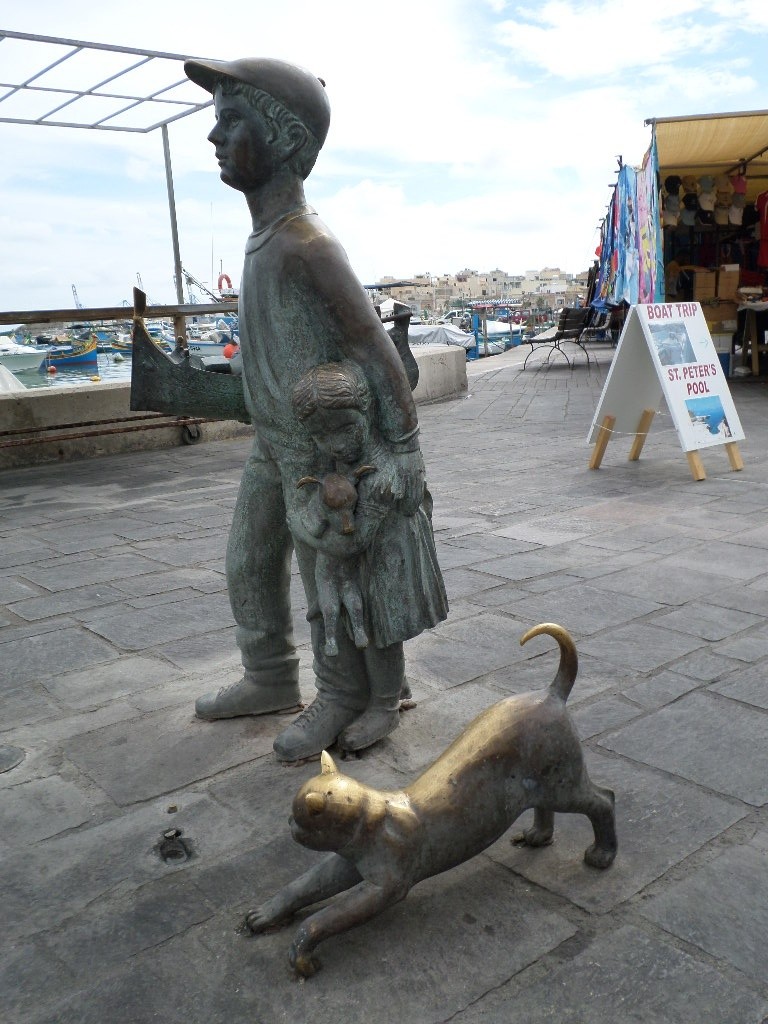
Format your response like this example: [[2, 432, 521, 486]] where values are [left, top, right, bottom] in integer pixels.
[[235, 622, 617, 977]]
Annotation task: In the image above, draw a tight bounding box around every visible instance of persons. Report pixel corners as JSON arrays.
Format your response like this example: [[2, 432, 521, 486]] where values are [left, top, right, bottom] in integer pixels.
[[292, 363, 452, 749], [183, 48, 424, 757]]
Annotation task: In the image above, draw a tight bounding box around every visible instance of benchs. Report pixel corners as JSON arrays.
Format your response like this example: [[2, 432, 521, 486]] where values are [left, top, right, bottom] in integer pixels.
[[522, 306, 613, 372]]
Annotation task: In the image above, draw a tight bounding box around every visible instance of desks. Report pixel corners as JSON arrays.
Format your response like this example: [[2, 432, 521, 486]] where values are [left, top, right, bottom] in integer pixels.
[[737, 301, 768, 377]]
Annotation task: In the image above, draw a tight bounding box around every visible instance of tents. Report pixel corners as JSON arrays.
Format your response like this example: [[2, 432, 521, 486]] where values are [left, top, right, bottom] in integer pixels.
[[586, 110, 768, 349]]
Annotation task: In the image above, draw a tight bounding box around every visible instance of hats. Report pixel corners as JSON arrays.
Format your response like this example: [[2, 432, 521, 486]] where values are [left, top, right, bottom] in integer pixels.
[[661, 172, 747, 227]]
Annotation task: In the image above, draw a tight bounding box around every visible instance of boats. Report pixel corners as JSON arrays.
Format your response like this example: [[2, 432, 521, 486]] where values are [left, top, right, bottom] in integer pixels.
[[408, 284, 585, 361], [0, 260, 242, 395]]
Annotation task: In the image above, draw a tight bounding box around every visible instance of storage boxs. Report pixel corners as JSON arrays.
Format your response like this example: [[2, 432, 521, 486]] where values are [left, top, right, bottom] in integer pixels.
[[665, 265, 740, 378]]
